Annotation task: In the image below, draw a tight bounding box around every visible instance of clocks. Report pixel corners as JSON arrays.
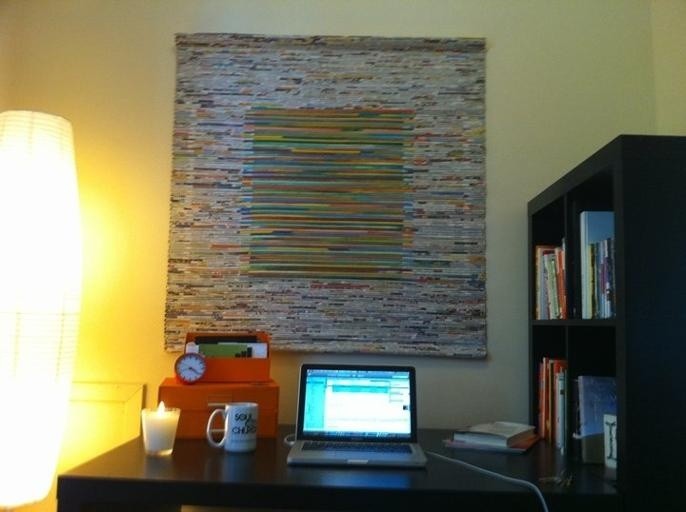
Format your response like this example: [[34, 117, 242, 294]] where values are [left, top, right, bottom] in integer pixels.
[[176, 352, 207, 385]]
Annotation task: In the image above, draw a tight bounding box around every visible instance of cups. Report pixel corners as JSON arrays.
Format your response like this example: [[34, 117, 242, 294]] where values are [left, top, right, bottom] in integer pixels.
[[141, 407, 182, 457], [206, 402, 258, 454]]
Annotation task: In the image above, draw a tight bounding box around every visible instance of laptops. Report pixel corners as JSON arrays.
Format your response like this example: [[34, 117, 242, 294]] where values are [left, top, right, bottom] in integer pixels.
[[286, 363, 429, 468]]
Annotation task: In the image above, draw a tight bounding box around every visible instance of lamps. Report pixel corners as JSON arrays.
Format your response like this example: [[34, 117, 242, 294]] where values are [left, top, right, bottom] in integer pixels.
[[2, 108, 79, 512]]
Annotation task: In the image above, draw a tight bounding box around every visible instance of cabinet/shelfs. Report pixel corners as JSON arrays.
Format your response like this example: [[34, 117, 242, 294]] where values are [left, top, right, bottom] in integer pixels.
[[526, 135, 686, 512]]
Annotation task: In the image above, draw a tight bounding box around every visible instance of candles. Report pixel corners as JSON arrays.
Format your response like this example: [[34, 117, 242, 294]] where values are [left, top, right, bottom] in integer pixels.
[[143, 401, 178, 450]]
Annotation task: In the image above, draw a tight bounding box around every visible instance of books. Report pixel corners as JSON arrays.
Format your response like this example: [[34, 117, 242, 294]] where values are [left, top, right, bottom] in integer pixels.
[[536, 355, 566, 455], [453, 421, 536, 447], [573, 375, 582, 460], [533, 211, 616, 320], [578, 375, 621, 465]]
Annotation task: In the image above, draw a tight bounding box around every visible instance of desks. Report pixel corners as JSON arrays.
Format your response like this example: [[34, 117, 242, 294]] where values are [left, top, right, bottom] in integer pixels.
[[56, 424, 617, 511]]
